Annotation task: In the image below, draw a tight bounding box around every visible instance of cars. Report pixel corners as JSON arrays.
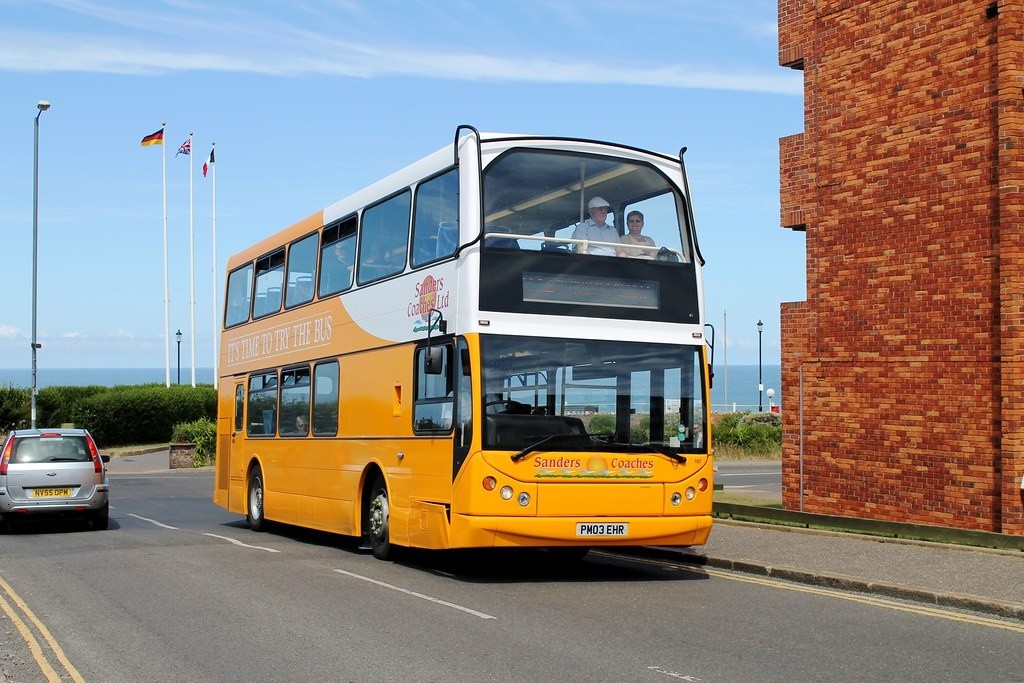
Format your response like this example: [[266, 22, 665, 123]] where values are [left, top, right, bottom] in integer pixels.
[[0, 426, 112, 532]]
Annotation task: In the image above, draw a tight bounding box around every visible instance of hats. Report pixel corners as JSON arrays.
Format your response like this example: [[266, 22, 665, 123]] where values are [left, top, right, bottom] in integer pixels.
[[587, 196, 609, 208]]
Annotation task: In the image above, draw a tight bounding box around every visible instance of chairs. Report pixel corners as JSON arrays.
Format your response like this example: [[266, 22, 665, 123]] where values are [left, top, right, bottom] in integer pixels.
[[228, 304, 239, 325], [73, 446, 85, 458], [281, 282, 296, 307], [485, 224, 520, 249], [263, 286, 281, 314], [293, 275, 315, 304], [239, 296, 251, 321], [436, 221, 459, 259], [540, 241, 569, 253], [254, 293, 267, 317], [414, 231, 438, 264]]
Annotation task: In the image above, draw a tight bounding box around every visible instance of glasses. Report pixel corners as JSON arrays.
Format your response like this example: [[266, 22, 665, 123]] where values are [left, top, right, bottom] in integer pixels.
[[629, 219, 641, 224]]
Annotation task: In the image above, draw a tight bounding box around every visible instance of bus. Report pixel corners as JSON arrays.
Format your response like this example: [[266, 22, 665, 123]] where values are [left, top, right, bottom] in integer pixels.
[[210, 124, 718, 562]]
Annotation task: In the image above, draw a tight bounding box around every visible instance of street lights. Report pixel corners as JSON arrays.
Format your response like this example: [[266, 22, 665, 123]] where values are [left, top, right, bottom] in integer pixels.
[[31, 100, 51, 432], [755, 320, 764, 413], [176, 329, 183, 385]]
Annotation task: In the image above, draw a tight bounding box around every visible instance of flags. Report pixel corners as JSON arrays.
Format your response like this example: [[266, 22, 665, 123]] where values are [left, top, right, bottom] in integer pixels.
[[141, 129, 163, 146], [203, 148, 214, 177], [175, 138, 190, 157]]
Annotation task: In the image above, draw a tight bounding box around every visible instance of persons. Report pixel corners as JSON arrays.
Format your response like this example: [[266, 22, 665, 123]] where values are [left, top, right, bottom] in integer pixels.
[[620, 211, 658, 260], [693, 424, 703, 448], [572, 197, 626, 257], [329, 239, 393, 291], [296, 416, 308, 432], [441, 365, 507, 429]]
